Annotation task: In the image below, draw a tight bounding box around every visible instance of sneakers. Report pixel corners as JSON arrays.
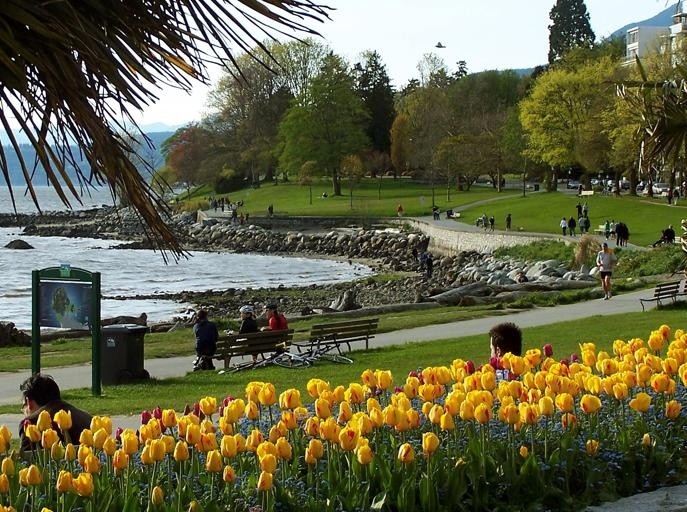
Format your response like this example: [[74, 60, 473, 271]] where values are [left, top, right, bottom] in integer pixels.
[[192, 360, 197, 365]]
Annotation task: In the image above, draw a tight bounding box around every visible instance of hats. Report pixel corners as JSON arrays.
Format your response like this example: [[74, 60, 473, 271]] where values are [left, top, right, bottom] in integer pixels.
[[240, 306, 253, 313], [263, 303, 277, 309]]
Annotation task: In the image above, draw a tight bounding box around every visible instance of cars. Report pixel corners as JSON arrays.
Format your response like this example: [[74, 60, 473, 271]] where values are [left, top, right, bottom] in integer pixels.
[[565, 178, 685, 198], [357, 168, 495, 185]]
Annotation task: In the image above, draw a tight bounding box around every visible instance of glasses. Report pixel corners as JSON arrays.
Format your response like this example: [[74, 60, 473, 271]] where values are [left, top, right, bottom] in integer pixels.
[[21, 403, 26, 413]]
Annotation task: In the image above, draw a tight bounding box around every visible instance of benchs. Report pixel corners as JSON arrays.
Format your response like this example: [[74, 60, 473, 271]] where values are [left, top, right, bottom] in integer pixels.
[[292, 318, 380, 355], [662, 237, 683, 245], [657, 192, 669, 198], [640, 190, 649, 197], [579, 191, 594, 199], [676, 280, 687, 295], [594, 225, 606, 236], [213, 329, 294, 369], [639, 281, 680, 311]]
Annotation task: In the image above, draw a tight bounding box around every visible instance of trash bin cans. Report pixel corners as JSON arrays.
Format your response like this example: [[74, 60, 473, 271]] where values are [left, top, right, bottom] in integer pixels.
[[446, 209, 453, 218], [98, 322, 150, 386], [534, 183, 539, 191]]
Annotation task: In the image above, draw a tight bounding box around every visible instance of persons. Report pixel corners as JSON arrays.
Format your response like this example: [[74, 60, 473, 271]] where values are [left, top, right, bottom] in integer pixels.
[[666, 187, 672, 203], [673, 189, 680, 205], [596, 243, 618, 300], [560, 202, 590, 237], [431, 205, 441, 221], [505, 213, 511, 229], [398, 204, 403, 218], [204, 196, 250, 226], [482, 214, 488, 231], [488, 215, 495, 231], [575, 184, 585, 198], [599, 180, 619, 197], [191, 304, 288, 370], [606, 219, 628, 247], [411, 247, 433, 278], [19, 375, 92, 451], [664, 224, 676, 242], [487, 324, 522, 360], [653, 230, 665, 247]]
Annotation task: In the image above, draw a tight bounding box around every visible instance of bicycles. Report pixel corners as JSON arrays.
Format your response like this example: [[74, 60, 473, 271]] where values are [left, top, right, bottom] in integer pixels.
[[219, 332, 356, 374]]
